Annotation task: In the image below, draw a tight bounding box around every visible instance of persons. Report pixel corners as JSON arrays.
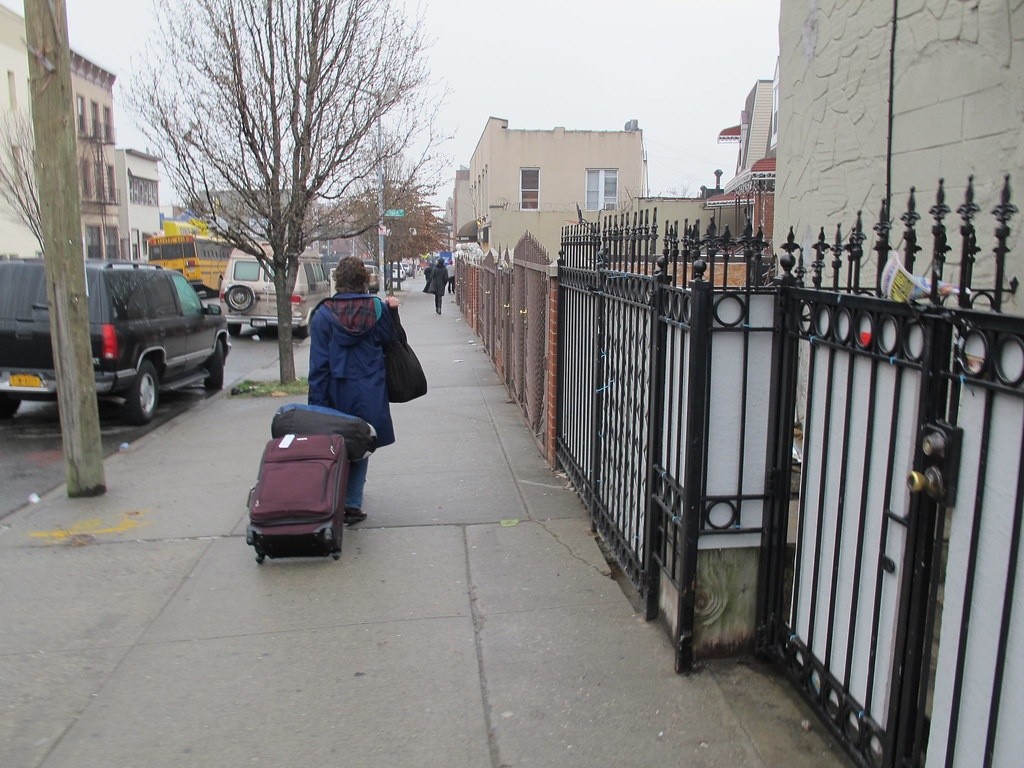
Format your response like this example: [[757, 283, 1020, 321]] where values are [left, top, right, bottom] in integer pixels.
[[424, 264, 432, 283], [307, 256, 401, 524], [430, 259, 448, 315], [446, 261, 455, 293]]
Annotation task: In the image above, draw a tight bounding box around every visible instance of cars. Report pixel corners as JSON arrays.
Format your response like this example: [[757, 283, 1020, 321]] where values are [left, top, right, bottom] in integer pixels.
[[363, 260, 412, 293]]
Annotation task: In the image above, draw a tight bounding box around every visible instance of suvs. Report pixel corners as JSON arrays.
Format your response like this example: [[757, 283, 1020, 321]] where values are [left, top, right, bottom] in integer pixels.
[[0, 257, 233, 426], [219, 240, 330, 339]]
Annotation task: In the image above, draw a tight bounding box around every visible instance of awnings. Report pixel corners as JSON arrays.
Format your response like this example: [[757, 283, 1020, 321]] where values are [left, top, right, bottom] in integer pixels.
[[456, 220, 477, 237]]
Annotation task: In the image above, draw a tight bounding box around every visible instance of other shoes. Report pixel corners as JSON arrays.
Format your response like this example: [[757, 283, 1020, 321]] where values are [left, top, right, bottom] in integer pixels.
[[436, 307, 441, 314], [343, 507, 367, 524]]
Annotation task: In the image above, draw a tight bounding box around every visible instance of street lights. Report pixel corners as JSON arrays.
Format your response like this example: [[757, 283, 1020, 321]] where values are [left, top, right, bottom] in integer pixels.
[[300, 71, 386, 295]]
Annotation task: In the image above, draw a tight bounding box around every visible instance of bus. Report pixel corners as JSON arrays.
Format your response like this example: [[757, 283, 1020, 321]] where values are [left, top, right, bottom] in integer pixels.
[[146, 235, 234, 298]]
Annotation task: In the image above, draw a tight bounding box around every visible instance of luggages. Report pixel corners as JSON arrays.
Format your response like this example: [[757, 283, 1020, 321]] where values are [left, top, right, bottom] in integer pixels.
[[246, 429, 349, 561]]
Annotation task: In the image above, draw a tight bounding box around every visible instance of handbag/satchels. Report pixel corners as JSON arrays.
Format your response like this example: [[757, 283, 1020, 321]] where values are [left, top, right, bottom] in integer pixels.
[[382, 304, 428, 403], [428, 276, 437, 293], [271, 399, 378, 461]]
